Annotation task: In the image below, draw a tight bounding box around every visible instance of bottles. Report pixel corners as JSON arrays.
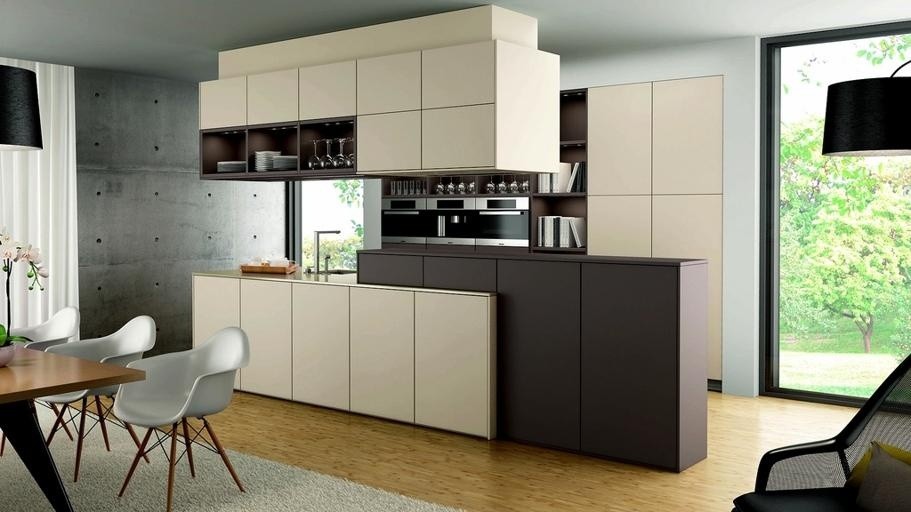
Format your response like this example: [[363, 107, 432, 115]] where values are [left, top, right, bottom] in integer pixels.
[[390, 181, 426, 195]]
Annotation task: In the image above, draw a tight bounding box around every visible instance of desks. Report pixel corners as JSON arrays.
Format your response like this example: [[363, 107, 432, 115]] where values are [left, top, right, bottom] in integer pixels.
[[0, 345, 146, 512]]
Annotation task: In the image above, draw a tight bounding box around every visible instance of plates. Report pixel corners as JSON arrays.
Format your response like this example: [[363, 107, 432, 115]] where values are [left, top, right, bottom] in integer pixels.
[[254, 150, 297, 173], [217, 160, 247, 174]]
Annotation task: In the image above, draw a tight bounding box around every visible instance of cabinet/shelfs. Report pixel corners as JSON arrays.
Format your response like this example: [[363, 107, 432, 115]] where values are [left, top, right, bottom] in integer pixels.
[[587, 196, 723, 395], [530, 89, 587, 255], [356, 250, 496, 293], [587, 73, 723, 196], [357, 39, 562, 177], [495, 259, 708, 472], [191, 275, 240, 390], [349, 285, 499, 441], [199, 62, 357, 180], [239, 278, 349, 412]]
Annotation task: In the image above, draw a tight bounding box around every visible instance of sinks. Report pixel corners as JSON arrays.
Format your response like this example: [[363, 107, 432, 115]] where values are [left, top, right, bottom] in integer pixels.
[[313, 265, 357, 276]]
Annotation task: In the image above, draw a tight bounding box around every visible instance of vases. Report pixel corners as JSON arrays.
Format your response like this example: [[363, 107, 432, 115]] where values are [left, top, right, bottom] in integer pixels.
[[0, 344, 15, 368]]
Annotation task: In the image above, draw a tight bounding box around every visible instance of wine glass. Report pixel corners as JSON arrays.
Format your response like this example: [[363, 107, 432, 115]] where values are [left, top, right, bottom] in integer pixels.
[[435, 176, 529, 196], [346, 136, 354, 169], [334, 137, 349, 169], [320, 139, 335, 169], [307, 139, 321, 170]]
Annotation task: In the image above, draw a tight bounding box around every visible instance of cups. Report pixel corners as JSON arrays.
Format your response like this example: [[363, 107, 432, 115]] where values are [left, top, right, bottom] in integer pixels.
[[246, 256, 265, 266]]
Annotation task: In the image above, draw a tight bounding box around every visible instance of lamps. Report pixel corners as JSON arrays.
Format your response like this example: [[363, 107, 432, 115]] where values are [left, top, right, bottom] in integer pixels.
[[822, 59, 911, 156], [0, 64, 43, 151]]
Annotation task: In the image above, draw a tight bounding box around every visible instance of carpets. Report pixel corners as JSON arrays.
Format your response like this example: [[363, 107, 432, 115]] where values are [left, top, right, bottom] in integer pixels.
[[0, 399, 474, 512]]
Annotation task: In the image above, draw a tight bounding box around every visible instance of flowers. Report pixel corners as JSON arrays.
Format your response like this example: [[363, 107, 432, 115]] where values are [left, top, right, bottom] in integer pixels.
[[0, 224, 49, 347]]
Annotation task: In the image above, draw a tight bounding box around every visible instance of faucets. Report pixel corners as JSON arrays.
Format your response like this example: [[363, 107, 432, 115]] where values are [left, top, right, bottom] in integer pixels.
[[322, 253, 333, 272], [313, 228, 342, 273]]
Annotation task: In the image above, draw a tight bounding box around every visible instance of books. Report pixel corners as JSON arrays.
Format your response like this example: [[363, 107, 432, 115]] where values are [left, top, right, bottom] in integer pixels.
[[537, 161, 585, 193], [537, 216, 586, 248]]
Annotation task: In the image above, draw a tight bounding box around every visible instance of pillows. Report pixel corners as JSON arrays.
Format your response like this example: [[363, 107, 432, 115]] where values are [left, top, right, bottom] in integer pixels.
[[843, 441, 911, 512]]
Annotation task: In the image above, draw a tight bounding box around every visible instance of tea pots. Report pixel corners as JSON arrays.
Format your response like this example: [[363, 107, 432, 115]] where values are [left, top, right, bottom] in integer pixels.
[[266, 257, 292, 267]]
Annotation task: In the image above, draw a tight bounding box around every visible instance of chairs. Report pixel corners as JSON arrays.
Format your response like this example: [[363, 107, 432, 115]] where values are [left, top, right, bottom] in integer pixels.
[[36, 315, 157, 482], [731, 354, 911, 512], [114, 327, 250, 512], [0, 306, 80, 457]]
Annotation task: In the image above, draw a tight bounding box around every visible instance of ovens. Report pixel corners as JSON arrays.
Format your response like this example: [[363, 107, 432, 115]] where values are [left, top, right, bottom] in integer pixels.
[[475, 196, 530, 247], [425, 198, 477, 246], [380, 199, 427, 245]]
